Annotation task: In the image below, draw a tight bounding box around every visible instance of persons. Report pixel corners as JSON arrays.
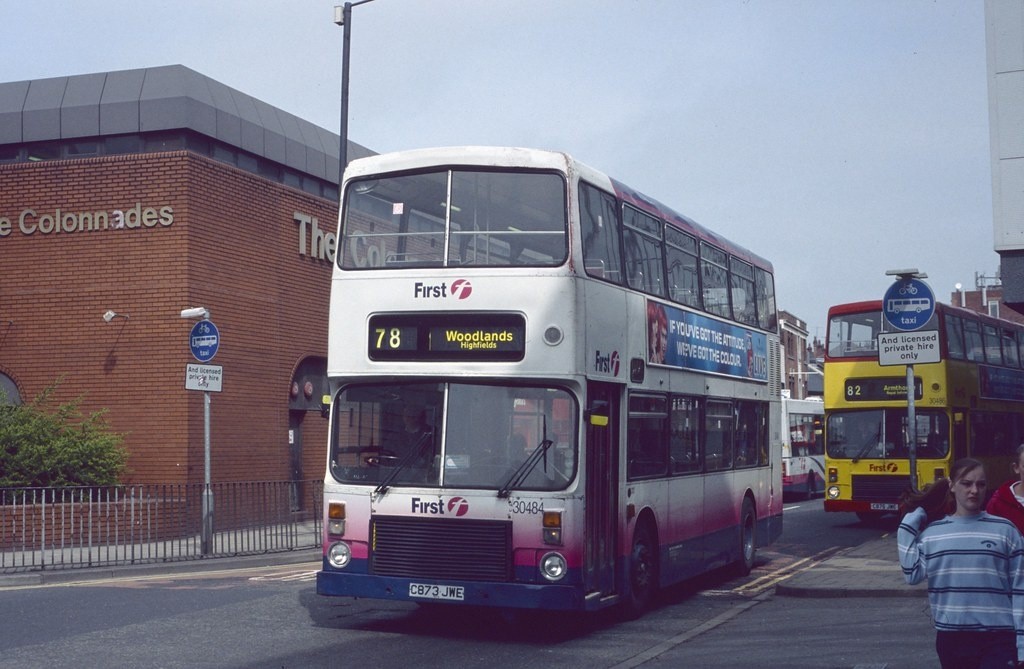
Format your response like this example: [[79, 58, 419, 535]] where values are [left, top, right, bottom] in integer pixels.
[[896, 458, 1024, 669], [854, 423, 877, 443], [987, 444, 1024, 536], [382, 403, 439, 466], [505, 434, 530, 463]]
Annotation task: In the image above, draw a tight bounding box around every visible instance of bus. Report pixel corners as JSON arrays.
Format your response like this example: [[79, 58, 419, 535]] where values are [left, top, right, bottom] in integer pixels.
[[782, 395, 844, 496], [313, 144, 785, 620], [812, 300, 1024, 524]]
